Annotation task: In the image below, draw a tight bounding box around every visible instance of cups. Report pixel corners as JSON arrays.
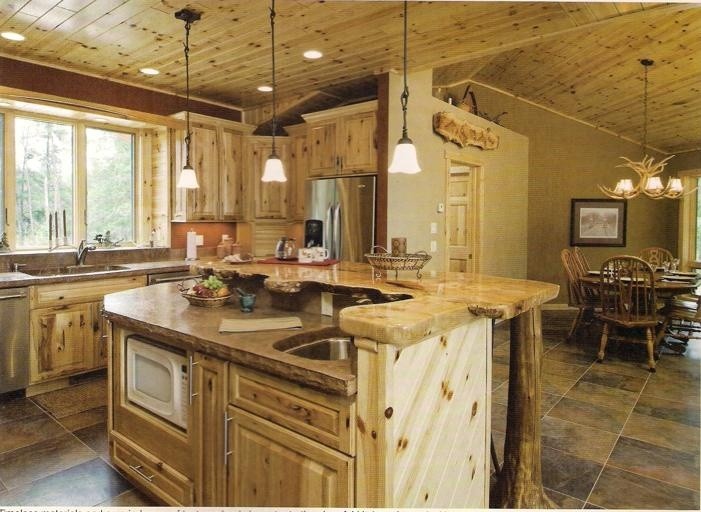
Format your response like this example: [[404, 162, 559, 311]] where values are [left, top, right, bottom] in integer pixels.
[[297, 248, 313, 263], [310, 247, 329, 263], [238, 293, 256, 313], [391, 236, 408, 258]]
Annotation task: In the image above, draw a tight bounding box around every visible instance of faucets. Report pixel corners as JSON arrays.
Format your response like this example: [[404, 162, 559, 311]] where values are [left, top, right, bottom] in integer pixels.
[[76, 239, 95, 267]]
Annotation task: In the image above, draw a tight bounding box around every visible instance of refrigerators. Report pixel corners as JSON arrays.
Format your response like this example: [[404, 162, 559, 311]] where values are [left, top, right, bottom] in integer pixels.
[[302, 175, 377, 261]]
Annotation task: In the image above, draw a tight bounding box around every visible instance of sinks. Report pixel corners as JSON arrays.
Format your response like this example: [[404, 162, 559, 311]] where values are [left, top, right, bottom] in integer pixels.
[[20, 265, 130, 277], [272, 325, 357, 362]]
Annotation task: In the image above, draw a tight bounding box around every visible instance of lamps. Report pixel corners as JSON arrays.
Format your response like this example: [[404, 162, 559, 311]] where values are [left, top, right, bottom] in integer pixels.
[[387, 1, 423, 175], [174, 8, 203, 188], [596, 56, 700, 204], [261, 1, 288, 182]]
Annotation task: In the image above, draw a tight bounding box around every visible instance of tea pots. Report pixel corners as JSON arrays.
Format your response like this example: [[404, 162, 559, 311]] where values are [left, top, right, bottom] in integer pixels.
[[273, 236, 296, 261]]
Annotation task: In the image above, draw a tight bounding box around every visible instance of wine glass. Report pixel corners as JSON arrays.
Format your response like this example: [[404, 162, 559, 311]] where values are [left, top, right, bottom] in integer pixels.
[[660, 256, 679, 273], [608, 260, 625, 279]]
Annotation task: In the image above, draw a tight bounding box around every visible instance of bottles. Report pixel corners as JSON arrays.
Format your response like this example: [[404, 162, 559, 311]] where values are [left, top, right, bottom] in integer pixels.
[[216, 237, 242, 259]]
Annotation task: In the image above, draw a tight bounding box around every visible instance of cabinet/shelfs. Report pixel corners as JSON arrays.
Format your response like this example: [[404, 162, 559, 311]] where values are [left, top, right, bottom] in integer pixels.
[[302, 100, 377, 180], [29, 276, 149, 384], [108, 433, 193, 507], [171, 111, 259, 223], [283, 123, 314, 222], [187, 349, 359, 508], [241, 136, 294, 222]]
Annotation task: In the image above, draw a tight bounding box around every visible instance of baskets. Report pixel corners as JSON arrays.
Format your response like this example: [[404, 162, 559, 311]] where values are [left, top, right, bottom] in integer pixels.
[[365, 246, 432, 270], [178, 277, 232, 307]]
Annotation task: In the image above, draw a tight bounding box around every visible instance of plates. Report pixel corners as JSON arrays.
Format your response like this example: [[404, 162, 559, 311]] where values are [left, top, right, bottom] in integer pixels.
[[223, 259, 250, 264], [586, 264, 697, 282]]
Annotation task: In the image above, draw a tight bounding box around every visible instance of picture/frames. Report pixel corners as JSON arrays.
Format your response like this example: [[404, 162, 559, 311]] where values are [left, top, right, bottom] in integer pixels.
[[570, 197, 627, 247]]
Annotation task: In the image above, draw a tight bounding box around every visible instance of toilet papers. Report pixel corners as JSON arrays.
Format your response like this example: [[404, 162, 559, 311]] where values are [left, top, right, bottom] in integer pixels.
[[186, 231, 197, 258]]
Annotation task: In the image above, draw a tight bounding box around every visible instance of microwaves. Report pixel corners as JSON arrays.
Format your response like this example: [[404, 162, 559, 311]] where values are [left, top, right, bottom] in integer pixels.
[[123, 336, 192, 434]]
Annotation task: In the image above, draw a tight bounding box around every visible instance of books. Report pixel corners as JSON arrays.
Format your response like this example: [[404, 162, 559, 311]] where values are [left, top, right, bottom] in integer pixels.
[[220, 317, 301, 333]]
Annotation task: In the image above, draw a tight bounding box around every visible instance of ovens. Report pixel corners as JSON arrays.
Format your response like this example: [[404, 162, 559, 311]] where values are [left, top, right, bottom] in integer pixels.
[[146, 273, 205, 285]]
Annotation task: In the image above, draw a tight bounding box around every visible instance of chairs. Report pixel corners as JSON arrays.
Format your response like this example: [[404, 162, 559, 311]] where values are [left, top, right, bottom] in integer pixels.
[[560, 246, 701, 371]]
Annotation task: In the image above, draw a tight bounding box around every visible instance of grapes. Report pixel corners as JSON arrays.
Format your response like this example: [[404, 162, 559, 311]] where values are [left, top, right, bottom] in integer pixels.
[[201, 275, 223, 289]]
